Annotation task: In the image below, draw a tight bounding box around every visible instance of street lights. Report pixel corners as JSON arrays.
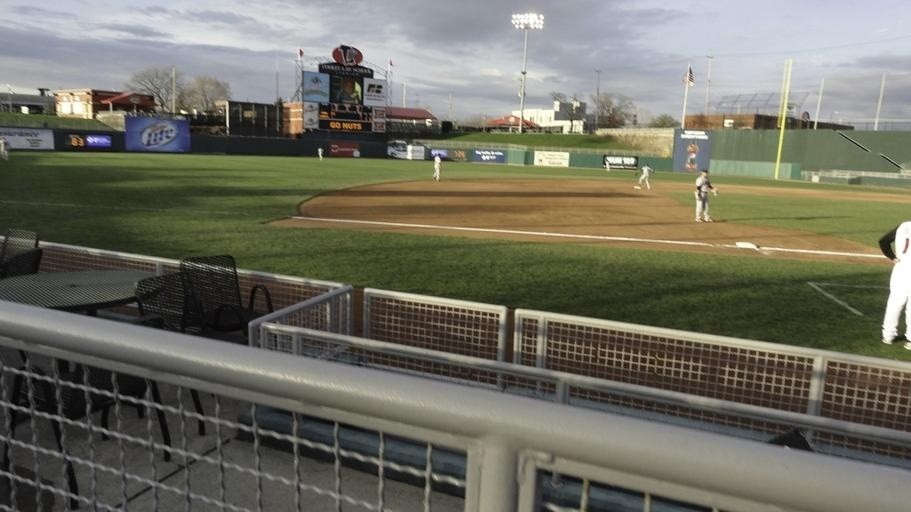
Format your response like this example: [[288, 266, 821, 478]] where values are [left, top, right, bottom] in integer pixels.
[[510, 12, 546, 133]]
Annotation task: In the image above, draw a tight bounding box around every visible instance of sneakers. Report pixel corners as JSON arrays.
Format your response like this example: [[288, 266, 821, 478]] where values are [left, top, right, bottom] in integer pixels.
[[882, 337, 893, 345], [903, 341, 911, 350], [696, 218, 714, 222]]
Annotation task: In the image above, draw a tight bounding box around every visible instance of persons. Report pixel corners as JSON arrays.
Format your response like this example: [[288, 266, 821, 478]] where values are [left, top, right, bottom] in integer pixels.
[[696, 168, 719, 222], [433, 153, 444, 182], [317, 146, 326, 161], [634, 163, 657, 190], [0, 136, 11, 161], [686, 138, 699, 173], [879, 217, 910, 345], [606, 158, 611, 171]]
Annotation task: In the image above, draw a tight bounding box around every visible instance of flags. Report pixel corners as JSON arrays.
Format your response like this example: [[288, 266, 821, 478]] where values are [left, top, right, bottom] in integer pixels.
[[682, 65, 697, 90]]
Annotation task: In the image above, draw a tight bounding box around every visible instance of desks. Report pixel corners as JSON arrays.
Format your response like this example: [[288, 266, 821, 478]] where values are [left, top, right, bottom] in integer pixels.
[[2, 269, 165, 440]]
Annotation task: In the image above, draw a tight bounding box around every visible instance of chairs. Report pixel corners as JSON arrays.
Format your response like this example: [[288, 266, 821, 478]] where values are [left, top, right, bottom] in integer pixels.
[[97, 270, 208, 461], [2, 229, 43, 261], [2, 347, 146, 496], [3, 249, 46, 277], [179, 253, 274, 339]]
